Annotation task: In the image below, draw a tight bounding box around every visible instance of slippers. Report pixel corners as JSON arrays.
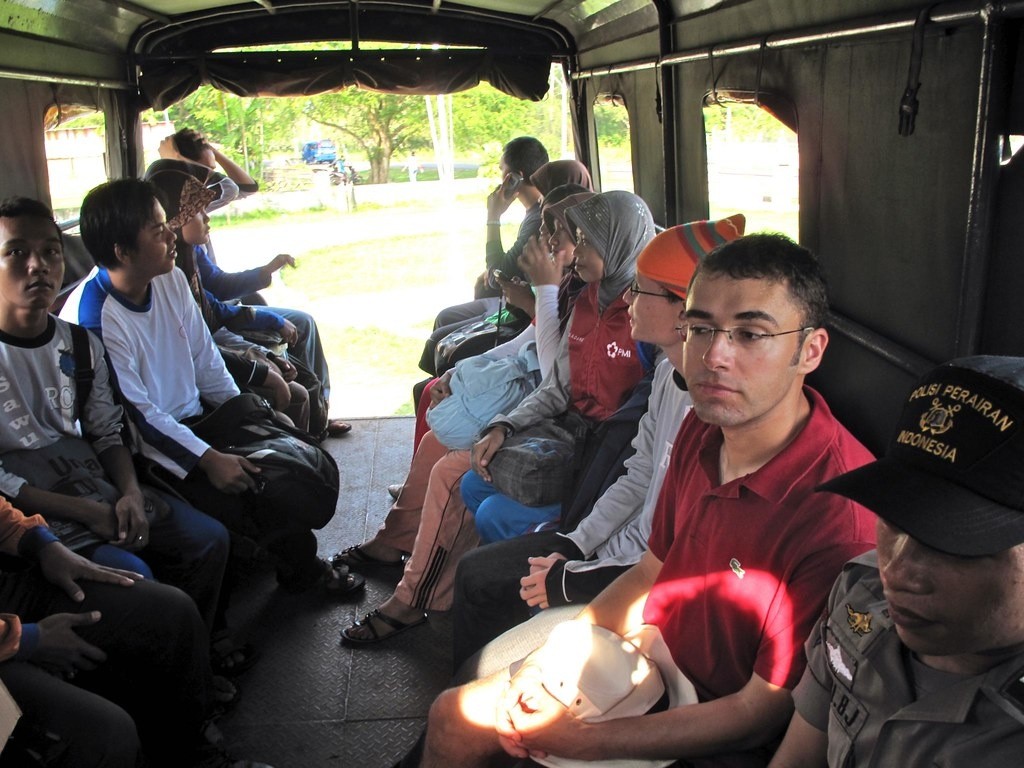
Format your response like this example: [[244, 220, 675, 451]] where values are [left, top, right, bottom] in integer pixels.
[[340, 609, 429, 646], [328, 543, 405, 570]]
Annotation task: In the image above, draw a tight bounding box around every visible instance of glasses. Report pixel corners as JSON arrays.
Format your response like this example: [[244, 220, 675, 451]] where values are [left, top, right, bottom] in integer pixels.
[[674, 322, 809, 349], [630, 280, 674, 301]]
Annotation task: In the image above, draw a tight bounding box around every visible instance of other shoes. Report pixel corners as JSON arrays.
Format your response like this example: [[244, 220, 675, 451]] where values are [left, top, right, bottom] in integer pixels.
[[201, 746, 274, 768], [389, 484, 404, 500]]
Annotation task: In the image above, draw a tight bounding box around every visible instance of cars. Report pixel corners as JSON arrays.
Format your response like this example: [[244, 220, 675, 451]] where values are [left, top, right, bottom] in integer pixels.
[[300, 139, 337, 165]]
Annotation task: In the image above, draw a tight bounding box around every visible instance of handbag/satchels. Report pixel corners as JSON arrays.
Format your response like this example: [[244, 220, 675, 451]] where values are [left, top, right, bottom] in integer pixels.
[[486, 409, 595, 507], [3, 438, 172, 550], [505, 624, 667, 721], [424, 339, 540, 451]]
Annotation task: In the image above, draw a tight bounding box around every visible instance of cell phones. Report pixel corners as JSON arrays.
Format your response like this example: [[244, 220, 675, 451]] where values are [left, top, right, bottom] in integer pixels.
[[493, 270, 512, 283], [504, 173, 524, 199], [244, 468, 271, 492]]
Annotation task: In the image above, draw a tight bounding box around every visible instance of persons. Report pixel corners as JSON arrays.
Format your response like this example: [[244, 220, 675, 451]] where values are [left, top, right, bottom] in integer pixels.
[[0, 131, 364, 768], [343, 135, 1024, 768]]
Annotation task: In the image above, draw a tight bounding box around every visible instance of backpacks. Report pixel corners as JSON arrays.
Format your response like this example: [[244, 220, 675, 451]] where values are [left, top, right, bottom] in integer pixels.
[[183, 394, 339, 529]]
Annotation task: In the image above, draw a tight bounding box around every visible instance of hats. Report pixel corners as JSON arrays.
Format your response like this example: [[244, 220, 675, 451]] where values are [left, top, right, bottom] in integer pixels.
[[635, 214, 746, 301], [810, 357, 1024, 555]]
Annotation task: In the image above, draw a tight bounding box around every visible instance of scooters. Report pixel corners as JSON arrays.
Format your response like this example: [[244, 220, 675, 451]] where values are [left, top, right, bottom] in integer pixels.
[[328, 166, 363, 186]]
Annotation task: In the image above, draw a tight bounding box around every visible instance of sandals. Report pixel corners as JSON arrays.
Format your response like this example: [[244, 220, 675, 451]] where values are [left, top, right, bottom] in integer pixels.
[[212, 629, 262, 676], [336, 572, 366, 595], [327, 418, 353, 435]]
